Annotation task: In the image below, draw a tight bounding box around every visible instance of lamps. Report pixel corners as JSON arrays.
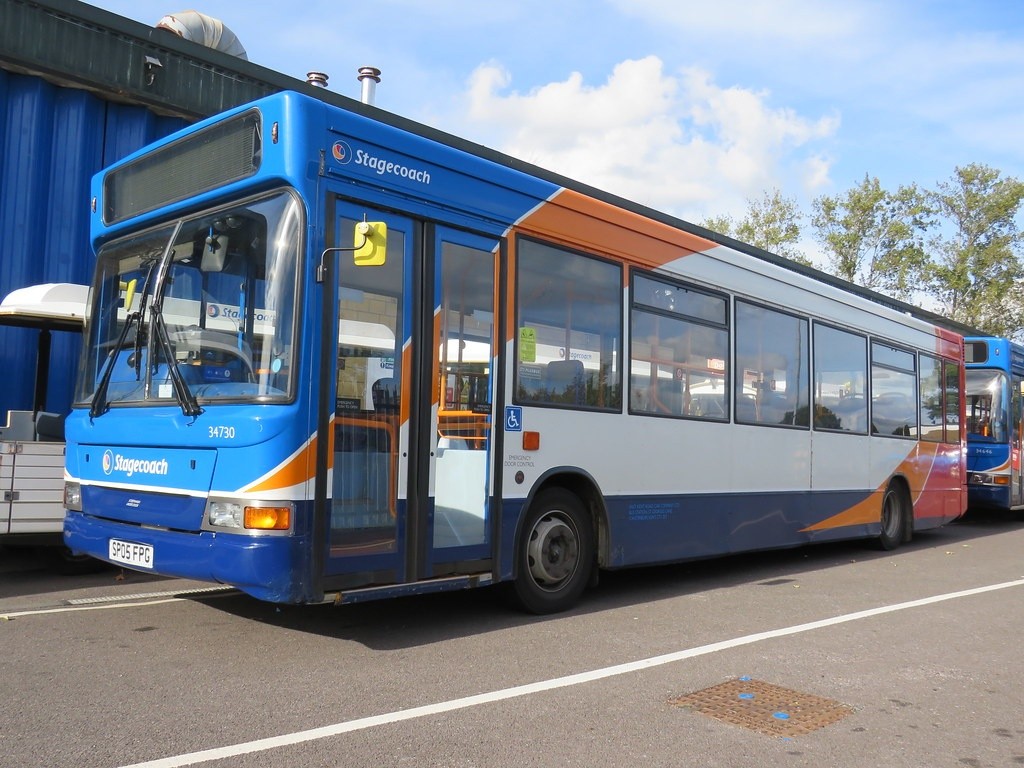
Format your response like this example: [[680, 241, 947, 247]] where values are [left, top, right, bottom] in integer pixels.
[[143, 55, 162, 85]]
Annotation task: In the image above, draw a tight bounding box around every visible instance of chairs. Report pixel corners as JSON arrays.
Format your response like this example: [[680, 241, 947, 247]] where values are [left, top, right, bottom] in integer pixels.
[[371, 376, 400, 415], [688, 393, 791, 422], [547, 359, 586, 407]]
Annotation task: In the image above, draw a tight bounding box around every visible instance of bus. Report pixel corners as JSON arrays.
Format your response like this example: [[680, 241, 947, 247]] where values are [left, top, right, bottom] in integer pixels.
[[62, 89, 969, 616], [963, 337, 1024, 522]]
